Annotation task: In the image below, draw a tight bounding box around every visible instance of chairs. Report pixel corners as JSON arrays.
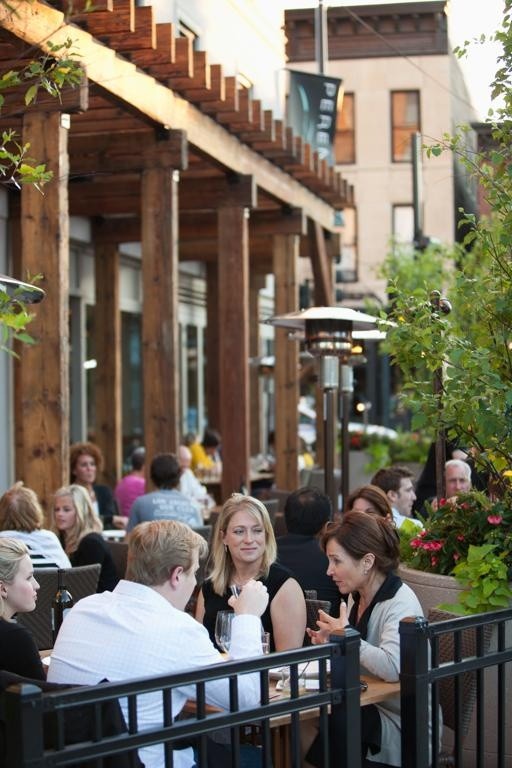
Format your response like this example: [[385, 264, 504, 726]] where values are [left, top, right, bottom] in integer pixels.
[[191, 487, 291, 544], [426, 608, 493, 759], [306, 589, 331, 644], [18, 563, 102, 651], [1, 672, 143, 766]]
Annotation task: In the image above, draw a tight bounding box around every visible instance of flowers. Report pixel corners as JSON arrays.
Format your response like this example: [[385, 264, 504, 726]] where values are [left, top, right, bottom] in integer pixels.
[[387, 480, 510, 582]]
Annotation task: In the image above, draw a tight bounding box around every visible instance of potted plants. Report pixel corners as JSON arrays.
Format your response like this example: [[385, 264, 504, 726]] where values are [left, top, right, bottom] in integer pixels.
[[356, 0, 512, 768]]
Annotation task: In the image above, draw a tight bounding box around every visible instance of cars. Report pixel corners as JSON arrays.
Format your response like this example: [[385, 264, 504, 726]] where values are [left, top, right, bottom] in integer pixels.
[[295, 398, 398, 454]]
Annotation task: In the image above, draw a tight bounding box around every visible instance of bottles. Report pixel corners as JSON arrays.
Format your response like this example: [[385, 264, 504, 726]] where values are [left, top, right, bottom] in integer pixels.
[[50, 567, 73, 652]]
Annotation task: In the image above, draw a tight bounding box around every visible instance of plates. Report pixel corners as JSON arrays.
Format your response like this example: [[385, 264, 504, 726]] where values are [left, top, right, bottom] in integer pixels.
[[271, 664, 328, 683]]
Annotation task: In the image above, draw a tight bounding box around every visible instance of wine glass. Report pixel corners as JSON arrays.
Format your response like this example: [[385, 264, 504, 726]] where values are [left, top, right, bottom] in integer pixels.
[[214, 581, 271, 688]]
